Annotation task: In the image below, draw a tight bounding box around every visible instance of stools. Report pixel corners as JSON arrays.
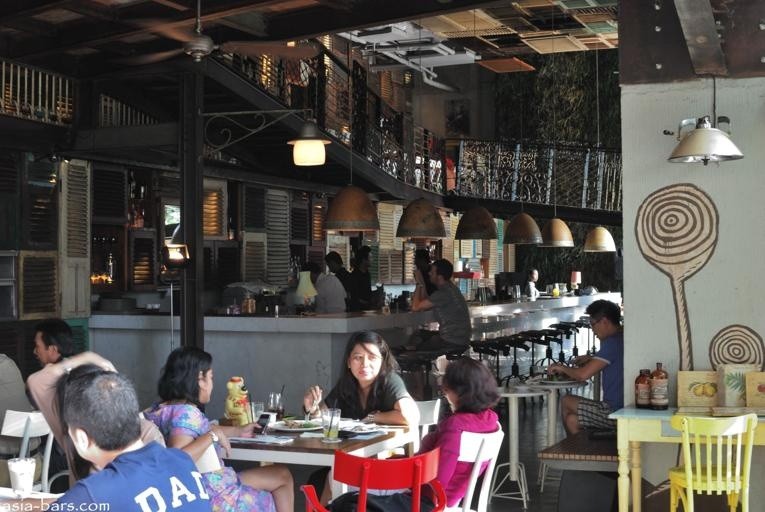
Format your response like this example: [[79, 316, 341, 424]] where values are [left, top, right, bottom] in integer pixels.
[[391, 314, 624, 432]]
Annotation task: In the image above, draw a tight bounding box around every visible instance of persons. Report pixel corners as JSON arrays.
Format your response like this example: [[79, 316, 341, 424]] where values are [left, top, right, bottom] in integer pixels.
[[24, 319, 79, 370], [544, 299, 623, 436], [302, 245, 472, 401], [139, 345, 297, 511], [0, 349, 44, 488], [299, 328, 424, 512], [45, 369, 213, 512], [0, 352, 168, 512], [523, 268, 541, 302], [326, 357, 499, 511]]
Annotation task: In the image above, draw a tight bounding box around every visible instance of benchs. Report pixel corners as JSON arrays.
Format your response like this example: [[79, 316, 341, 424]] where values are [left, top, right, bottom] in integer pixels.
[[535, 426, 618, 494]]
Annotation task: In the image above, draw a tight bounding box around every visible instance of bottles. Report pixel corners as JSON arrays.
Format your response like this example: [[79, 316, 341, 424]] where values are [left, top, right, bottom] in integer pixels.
[[635, 368, 651, 409], [650, 363, 669, 410]]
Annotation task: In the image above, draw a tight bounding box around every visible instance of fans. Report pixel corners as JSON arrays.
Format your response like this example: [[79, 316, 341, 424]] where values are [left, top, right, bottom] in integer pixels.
[[113, 17, 321, 128]]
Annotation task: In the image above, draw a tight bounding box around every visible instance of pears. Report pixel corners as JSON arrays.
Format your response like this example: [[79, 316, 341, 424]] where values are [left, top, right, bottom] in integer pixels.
[[704, 384, 715, 397], [693, 384, 703, 396]]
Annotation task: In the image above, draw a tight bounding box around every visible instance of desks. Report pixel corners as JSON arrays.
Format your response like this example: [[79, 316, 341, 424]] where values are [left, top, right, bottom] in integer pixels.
[[605, 405, 765, 512]]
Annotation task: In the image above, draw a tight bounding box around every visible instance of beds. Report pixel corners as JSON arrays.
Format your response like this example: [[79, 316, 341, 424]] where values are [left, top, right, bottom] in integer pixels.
[[488, 370, 601, 511]]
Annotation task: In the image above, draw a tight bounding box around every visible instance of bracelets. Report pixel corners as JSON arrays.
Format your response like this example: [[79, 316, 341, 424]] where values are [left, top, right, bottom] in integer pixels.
[[367, 408, 380, 422], [208, 431, 218, 444]]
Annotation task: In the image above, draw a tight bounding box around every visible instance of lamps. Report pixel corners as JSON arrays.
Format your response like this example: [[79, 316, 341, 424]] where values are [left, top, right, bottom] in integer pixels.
[[295, 271, 319, 316], [667, 72, 745, 166], [570, 271, 582, 296]]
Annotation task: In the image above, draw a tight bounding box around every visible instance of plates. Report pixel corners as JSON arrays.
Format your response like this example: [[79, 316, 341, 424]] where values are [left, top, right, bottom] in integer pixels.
[[543, 380, 577, 384], [363, 310, 376, 314], [339, 426, 378, 434], [270, 420, 323, 431], [312, 416, 356, 423]]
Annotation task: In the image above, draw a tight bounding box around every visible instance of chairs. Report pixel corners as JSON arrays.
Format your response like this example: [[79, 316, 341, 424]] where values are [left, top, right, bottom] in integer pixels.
[[666, 412, 757, 512], [299, 398, 506, 512], [0, 407, 222, 493]]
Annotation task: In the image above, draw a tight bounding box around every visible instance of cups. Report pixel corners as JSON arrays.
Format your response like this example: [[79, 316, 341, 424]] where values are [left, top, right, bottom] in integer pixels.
[[268, 391, 286, 420], [250, 402, 264, 422], [320, 408, 341, 441]]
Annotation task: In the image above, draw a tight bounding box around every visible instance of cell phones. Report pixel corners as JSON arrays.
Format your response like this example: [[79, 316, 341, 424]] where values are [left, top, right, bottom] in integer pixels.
[[253, 413, 272, 434]]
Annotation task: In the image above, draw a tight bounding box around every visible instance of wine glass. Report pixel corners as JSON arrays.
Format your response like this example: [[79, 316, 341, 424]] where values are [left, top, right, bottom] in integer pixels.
[[8, 458, 37, 510]]
